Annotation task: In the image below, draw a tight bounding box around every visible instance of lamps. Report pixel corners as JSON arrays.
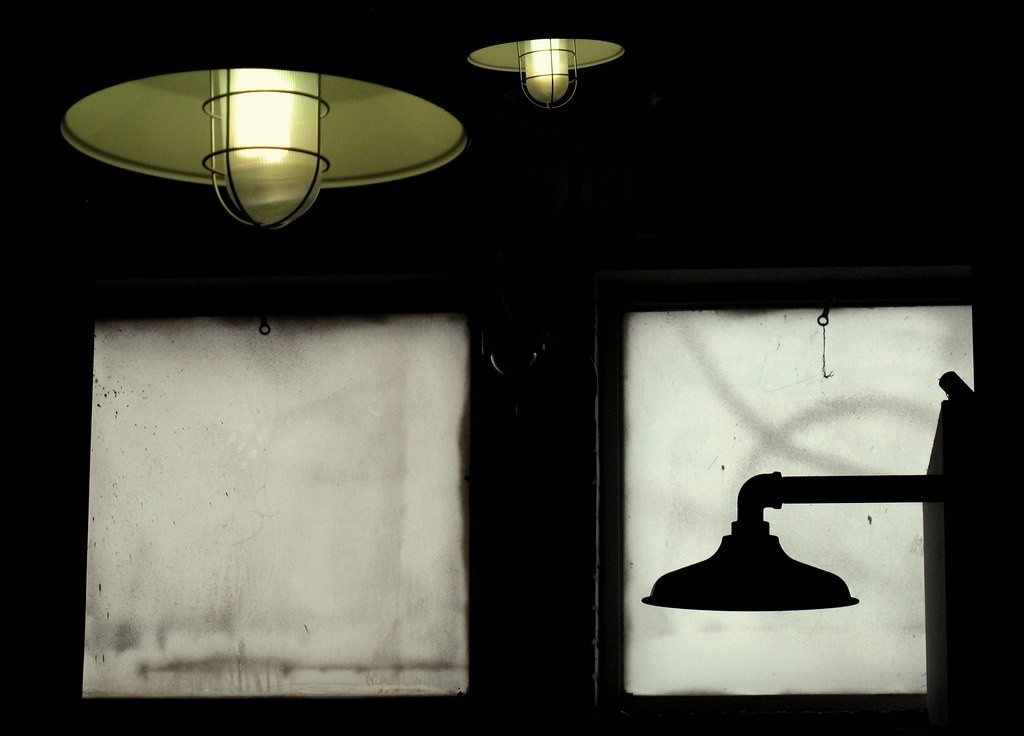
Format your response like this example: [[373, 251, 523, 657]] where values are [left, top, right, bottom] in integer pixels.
[[467, 0, 629, 108], [55, 0, 468, 229]]
